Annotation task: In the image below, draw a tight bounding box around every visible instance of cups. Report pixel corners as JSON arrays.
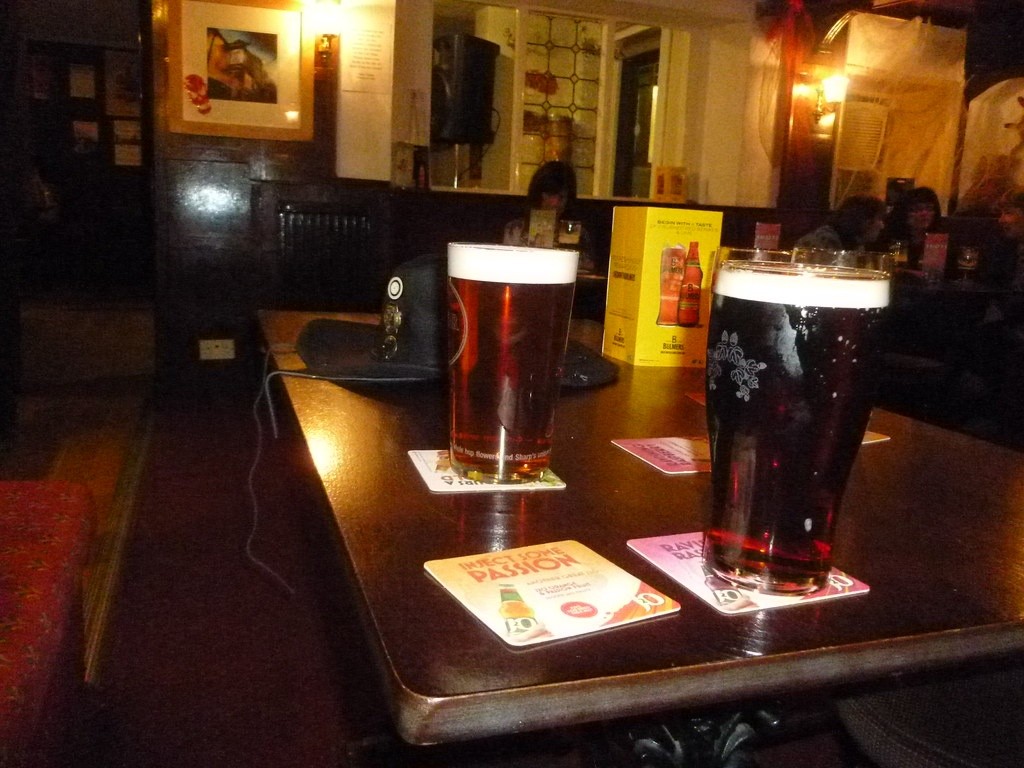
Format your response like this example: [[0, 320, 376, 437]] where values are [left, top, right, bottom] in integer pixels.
[[888, 240, 909, 270], [655, 249, 686, 325], [704, 248, 890, 593], [558, 219, 581, 249], [446, 244, 578, 481], [953, 240, 980, 279]]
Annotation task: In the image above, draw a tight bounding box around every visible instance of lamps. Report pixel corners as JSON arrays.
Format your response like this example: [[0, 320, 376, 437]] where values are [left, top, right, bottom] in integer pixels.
[[316, 3, 343, 83], [812, 68, 850, 126]]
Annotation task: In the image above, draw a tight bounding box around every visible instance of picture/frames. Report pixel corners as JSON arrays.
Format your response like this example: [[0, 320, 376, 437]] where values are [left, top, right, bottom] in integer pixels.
[[165, 0, 315, 142]]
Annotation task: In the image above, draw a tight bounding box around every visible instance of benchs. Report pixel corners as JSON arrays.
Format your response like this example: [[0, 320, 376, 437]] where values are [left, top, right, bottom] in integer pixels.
[[0, 298, 165, 768]]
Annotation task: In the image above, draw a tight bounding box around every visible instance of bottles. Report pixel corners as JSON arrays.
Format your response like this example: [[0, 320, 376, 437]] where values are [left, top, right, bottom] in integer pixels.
[[677, 242, 702, 326]]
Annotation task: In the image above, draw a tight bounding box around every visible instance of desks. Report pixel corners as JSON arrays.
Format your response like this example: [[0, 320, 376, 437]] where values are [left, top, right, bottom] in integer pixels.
[[253, 308, 1024, 768]]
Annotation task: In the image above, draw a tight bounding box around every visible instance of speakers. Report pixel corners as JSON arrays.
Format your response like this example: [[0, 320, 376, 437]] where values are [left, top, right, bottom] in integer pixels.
[[431, 31, 500, 144]]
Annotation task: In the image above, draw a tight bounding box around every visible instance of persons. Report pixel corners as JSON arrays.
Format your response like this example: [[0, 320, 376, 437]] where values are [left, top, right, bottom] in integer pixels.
[[503, 161, 597, 316], [790, 187, 1024, 361]]
